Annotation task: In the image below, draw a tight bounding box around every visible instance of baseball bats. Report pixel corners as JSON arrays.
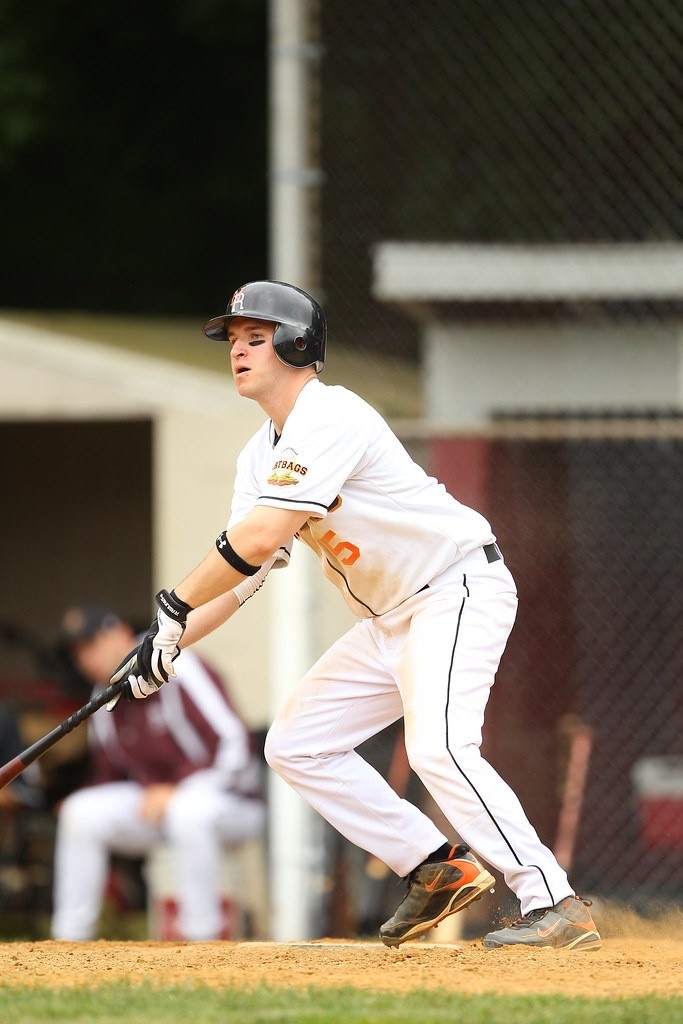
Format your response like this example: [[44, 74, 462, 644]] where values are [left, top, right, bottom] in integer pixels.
[[0, 660, 139, 788]]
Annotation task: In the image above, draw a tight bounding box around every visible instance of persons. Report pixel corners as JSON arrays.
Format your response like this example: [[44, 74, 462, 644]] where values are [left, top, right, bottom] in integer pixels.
[[107, 281, 604, 952], [0, 604, 266, 944]]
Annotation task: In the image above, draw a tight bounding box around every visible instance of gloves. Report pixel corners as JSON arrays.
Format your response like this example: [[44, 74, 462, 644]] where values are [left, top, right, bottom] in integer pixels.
[[136, 588, 195, 683], [105, 643, 181, 712]]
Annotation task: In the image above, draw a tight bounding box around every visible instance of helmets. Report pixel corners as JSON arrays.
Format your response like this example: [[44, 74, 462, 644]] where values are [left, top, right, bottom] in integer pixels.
[[203, 279, 326, 374]]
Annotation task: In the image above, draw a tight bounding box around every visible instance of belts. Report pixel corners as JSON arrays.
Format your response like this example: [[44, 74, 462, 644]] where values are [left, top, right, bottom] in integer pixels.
[[418, 545, 501, 595]]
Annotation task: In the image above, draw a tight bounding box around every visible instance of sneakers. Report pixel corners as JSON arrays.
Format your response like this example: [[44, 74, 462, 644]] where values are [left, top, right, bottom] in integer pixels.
[[482, 895, 603, 952], [378, 843, 497, 949]]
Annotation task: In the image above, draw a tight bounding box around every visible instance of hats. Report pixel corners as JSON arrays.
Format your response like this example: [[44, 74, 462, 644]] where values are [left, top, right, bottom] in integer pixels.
[[60, 605, 117, 661]]
